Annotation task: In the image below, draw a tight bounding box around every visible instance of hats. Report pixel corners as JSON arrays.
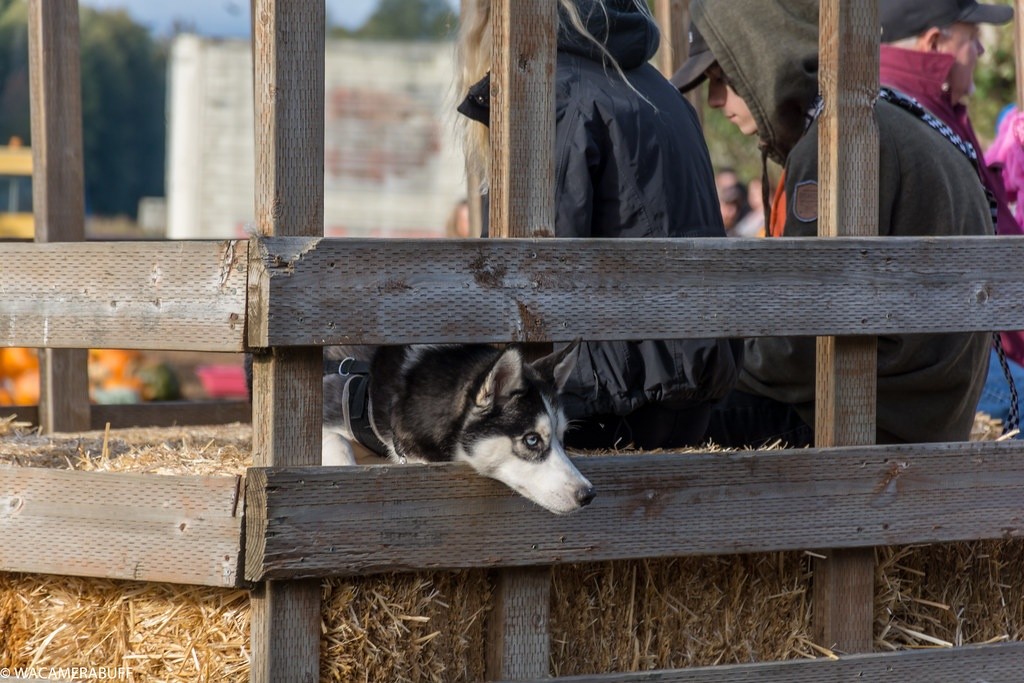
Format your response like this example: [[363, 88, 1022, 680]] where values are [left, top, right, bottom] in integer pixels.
[[669, 20, 716, 94], [879, 0, 1014, 43]]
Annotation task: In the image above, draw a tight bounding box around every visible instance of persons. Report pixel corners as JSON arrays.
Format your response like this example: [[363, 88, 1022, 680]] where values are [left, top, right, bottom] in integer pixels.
[[715, 166, 774, 236], [452, 0, 743, 449], [878, 0, 1024, 439], [669, 0, 995, 444]]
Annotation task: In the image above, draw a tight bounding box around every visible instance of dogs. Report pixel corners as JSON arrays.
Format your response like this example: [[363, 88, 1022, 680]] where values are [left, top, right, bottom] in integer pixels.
[[242, 344, 598, 518]]
